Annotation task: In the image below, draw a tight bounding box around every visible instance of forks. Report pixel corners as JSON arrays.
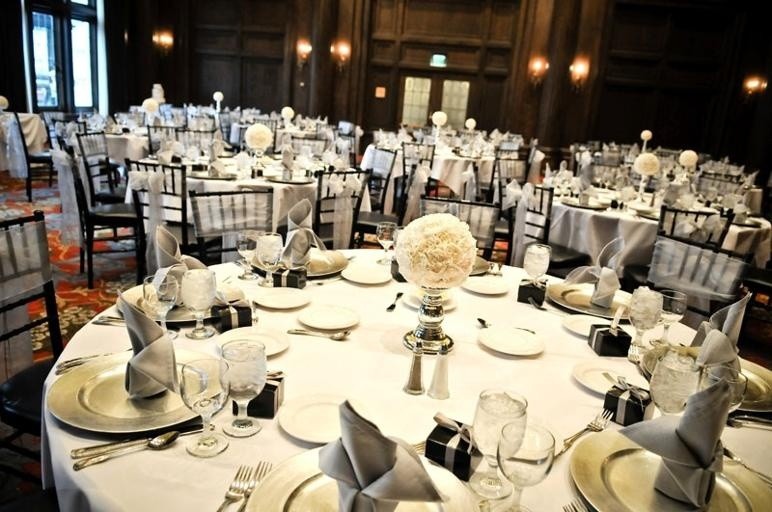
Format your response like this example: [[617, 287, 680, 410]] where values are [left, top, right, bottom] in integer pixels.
[[554, 408, 614, 456], [236, 461, 273, 512], [215, 463, 254, 512], [627, 344, 651, 387], [563, 497, 588, 512]]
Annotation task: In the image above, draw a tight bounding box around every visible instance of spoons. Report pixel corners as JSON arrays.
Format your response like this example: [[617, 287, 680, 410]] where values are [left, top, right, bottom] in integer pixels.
[[720, 446, 772, 484], [72, 429, 178, 471], [286, 328, 351, 341], [476, 318, 535, 333]]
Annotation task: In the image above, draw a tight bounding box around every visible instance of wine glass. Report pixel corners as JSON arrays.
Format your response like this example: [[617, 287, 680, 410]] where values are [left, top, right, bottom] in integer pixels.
[[219, 339, 268, 438], [142, 274, 178, 339], [642, 345, 772, 414], [490, 421, 555, 512], [522, 243, 552, 281], [648, 289, 688, 345], [629, 287, 664, 347], [179, 357, 231, 459], [375, 221, 397, 252], [649, 355, 700, 415], [234, 228, 264, 279], [256, 232, 284, 286], [180, 268, 217, 339], [701, 365, 748, 415], [469, 386, 528, 500]]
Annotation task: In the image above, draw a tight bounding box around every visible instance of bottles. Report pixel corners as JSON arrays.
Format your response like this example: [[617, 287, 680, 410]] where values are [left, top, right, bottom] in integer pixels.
[[427, 345, 451, 400], [404, 341, 425, 395]]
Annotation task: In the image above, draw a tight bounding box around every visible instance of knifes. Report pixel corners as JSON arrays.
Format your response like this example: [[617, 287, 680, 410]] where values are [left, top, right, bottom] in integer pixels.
[[70, 423, 216, 458]]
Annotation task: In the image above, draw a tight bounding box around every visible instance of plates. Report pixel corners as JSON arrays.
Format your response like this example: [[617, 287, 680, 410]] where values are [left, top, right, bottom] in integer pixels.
[[297, 305, 360, 330], [548, 281, 631, 320], [251, 250, 349, 277], [186, 173, 237, 180], [267, 175, 314, 185], [469, 256, 489, 277], [568, 428, 772, 512], [572, 359, 650, 396], [559, 198, 610, 211], [478, 326, 545, 358], [46, 345, 225, 435], [279, 391, 364, 444], [115, 281, 246, 323], [402, 292, 457, 312], [464, 277, 509, 296], [637, 210, 661, 221], [242, 443, 482, 512], [732, 218, 762, 228], [252, 287, 311, 309], [341, 265, 393, 285], [562, 313, 611, 338]]
